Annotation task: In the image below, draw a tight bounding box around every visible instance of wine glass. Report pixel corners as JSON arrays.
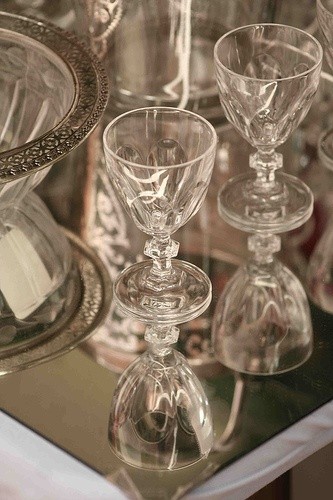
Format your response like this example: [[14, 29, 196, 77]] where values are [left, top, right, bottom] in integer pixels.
[[214, 23, 324, 227], [217, 203, 313, 375], [108, 294, 214, 472], [102, 106, 217, 319], [316, 0, 333, 163], [305, 152, 333, 314]]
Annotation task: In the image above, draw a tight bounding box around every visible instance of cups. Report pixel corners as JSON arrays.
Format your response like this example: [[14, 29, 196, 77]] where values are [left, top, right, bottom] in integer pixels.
[[72, 0, 274, 124]]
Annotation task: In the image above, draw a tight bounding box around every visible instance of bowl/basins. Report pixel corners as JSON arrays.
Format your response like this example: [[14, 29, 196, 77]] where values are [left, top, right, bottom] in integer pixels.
[[0, 8, 111, 212], [0, 191, 113, 376]]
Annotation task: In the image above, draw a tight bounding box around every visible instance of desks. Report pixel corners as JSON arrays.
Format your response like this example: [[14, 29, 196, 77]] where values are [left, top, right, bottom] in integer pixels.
[[0, 0, 333, 500]]
